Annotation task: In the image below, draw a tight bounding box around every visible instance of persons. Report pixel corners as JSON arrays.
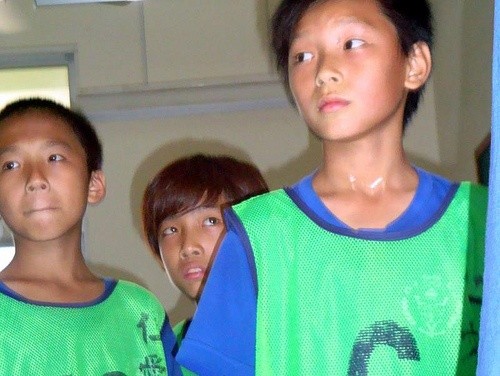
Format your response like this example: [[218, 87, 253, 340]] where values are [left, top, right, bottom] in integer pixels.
[[0, 99, 178, 376], [169, 0, 489, 375], [141, 156, 269, 347]]
